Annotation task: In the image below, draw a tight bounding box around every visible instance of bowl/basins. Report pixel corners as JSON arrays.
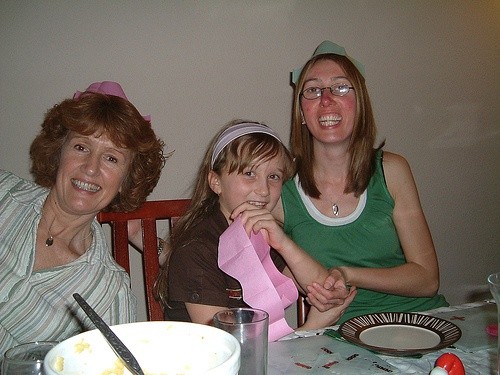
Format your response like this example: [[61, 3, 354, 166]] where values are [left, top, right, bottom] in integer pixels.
[[43, 321, 241, 375]]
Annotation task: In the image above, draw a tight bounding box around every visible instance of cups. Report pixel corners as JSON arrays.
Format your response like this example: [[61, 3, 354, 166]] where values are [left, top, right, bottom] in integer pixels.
[[213, 308, 270, 375], [1, 341, 63, 375]]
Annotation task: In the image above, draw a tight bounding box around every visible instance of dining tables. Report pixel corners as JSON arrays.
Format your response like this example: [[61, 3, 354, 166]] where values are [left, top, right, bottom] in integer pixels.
[[240, 298, 500, 375]]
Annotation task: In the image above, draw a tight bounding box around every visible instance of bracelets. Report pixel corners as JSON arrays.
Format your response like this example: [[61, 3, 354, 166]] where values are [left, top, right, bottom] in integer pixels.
[[157, 239, 165, 256]]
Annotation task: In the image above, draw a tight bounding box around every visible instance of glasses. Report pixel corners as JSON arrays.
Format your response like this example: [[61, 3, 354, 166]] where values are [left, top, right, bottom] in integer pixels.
[[302, 83, 354, 100]]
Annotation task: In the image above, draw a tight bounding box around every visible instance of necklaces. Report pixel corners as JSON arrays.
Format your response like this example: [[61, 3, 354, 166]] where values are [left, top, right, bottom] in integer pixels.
[[46, 216, 68, 246], [42, 212, 92, 241], [330, 201, 340, 217]]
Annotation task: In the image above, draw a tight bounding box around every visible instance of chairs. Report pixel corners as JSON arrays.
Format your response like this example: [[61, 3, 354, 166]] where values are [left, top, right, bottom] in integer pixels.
[[96, 199, 311, 329]]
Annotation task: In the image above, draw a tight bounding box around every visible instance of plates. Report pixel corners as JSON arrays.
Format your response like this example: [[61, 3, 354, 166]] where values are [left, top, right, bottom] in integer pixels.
[[338, 312, 462, 356]]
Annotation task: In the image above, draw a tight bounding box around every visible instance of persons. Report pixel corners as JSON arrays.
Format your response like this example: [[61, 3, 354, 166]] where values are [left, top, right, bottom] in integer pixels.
[[0, 81, 166, 375], [152, 119, 358, 334], [111, 40, 450, 327]]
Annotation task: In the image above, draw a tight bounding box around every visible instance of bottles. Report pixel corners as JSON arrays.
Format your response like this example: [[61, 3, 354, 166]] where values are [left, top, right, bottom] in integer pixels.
[[488, 273, 500, 375]]
